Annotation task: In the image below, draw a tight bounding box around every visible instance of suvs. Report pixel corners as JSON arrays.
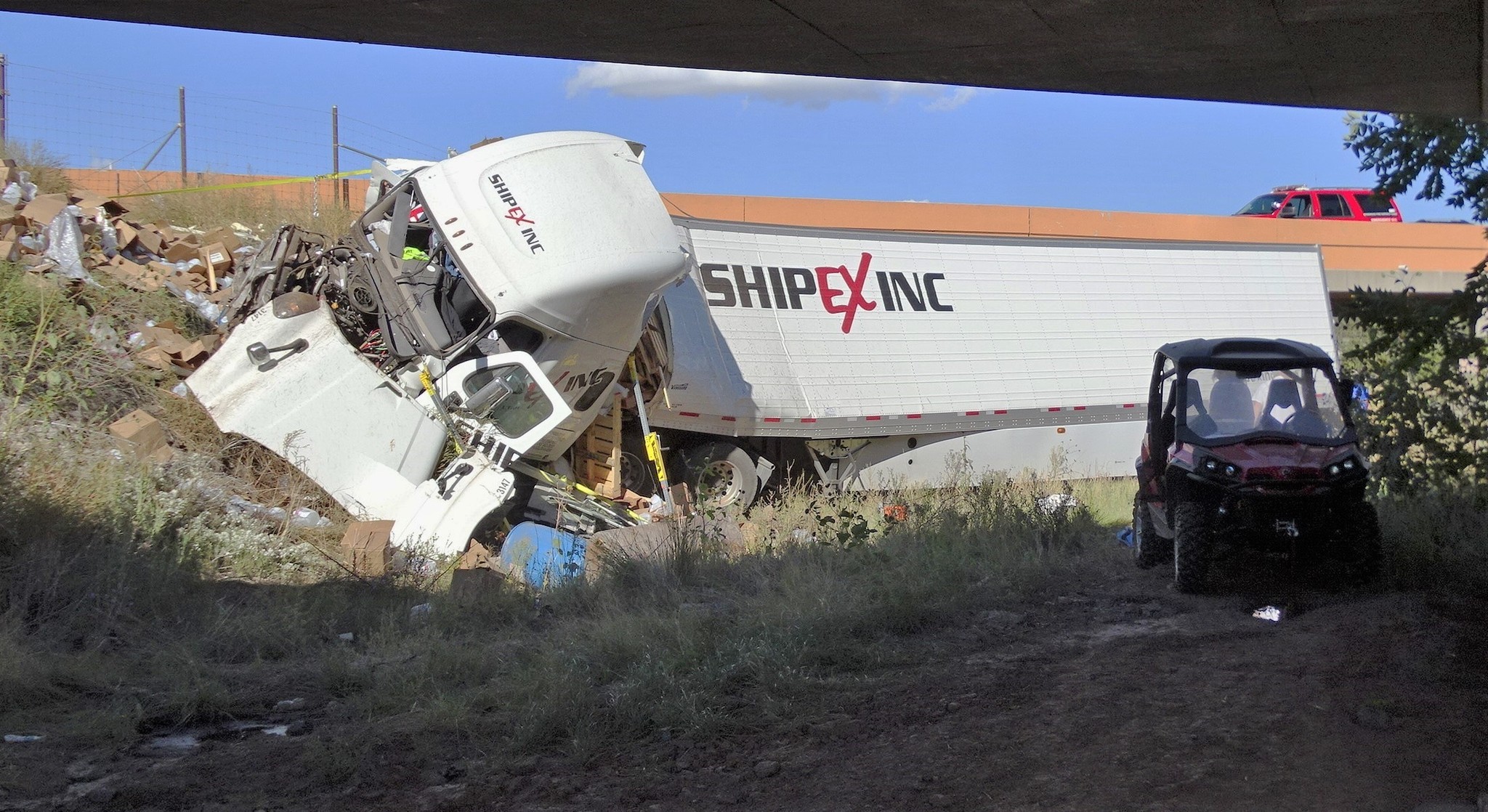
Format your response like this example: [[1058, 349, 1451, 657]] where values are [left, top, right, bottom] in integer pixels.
[[1134, 332, 1385, 592], [1233, 184, 1401, 222]]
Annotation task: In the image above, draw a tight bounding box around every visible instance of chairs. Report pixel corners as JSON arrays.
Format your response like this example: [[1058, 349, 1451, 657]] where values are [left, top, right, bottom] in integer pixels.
[[1161, 380, 1219, 447], [1256, 379, 1301, 433]]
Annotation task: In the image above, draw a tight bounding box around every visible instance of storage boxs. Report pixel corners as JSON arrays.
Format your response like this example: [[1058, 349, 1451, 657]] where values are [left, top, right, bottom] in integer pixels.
[[108, 408, 176, 469], [0, 158, 258, 307], [450, 541, 506, 613], [133, 320, 212, 376], [340, 519, 396, 578]]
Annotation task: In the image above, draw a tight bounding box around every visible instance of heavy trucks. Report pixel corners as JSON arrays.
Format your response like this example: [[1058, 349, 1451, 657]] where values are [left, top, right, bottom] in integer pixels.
[[181, 129, 1342, 557]]
